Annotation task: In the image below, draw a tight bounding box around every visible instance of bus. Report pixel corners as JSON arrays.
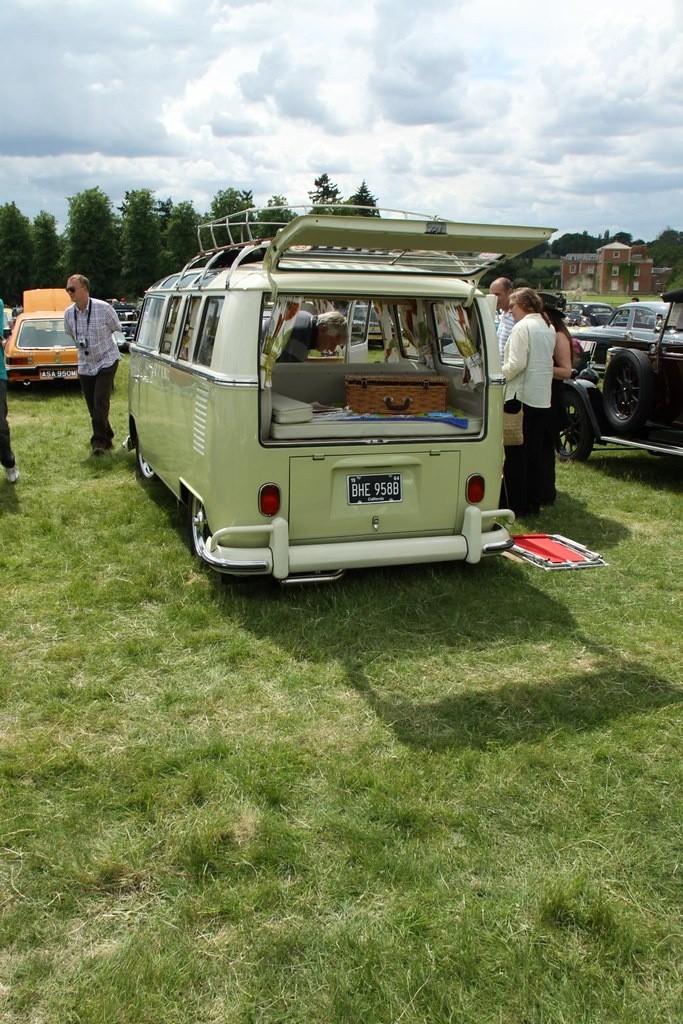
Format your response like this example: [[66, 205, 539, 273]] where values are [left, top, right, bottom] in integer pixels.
[[124, 199, 557, 584]]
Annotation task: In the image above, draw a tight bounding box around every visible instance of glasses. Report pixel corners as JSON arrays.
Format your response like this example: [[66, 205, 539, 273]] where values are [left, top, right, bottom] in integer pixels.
[[509, 301, 518, 310], [66, 285, 84, 293]]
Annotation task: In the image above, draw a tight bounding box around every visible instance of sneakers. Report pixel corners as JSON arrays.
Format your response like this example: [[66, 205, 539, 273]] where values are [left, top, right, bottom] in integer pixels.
[[6, 467, 19, 482]]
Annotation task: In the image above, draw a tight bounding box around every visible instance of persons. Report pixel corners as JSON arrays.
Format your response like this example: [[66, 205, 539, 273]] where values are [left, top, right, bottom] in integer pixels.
[[0, 299, 20, 483], [539, 303, 574, 506], [63, 275, 122, 454], [4, 302, 24, 339], [500, 287, 557, 518], [490, 277, 516, 368], [262, 310, 349, 362]]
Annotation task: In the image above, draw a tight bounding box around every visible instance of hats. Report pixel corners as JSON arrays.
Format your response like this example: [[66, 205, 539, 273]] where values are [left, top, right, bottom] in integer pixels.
[[536, 291, 567, 319]]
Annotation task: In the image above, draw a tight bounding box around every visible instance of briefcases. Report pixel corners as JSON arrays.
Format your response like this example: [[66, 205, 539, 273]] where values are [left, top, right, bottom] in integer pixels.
[[344, 368, 448, 414]]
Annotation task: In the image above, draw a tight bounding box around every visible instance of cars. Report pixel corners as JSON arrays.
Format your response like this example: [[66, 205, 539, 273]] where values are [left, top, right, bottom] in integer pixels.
[[6, 287, 80, 384], [557, 286, 683, 464], [3, 307, 15, 336], [106, 298, 140, 352]]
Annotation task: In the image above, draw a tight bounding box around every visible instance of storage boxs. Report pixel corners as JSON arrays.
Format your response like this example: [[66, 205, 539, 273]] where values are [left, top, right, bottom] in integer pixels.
[[342, 373, 451, 414]]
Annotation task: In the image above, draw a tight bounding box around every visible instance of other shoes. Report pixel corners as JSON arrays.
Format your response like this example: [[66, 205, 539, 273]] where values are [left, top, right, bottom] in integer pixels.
[[93, 444, 104, 455], [92, 441, 113, 449]]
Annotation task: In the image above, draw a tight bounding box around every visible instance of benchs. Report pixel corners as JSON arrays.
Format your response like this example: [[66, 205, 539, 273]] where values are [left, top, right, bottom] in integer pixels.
[[271, 361, 437, 406], [270, 405, 483, 439]]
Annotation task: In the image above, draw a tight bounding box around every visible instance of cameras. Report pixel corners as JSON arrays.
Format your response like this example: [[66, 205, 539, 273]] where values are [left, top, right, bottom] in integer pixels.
[[79, 339, 87, 348]]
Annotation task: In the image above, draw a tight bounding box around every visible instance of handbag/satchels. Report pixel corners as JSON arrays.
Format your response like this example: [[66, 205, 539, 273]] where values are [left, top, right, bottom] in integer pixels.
[[503, 410, 523, 445]]
